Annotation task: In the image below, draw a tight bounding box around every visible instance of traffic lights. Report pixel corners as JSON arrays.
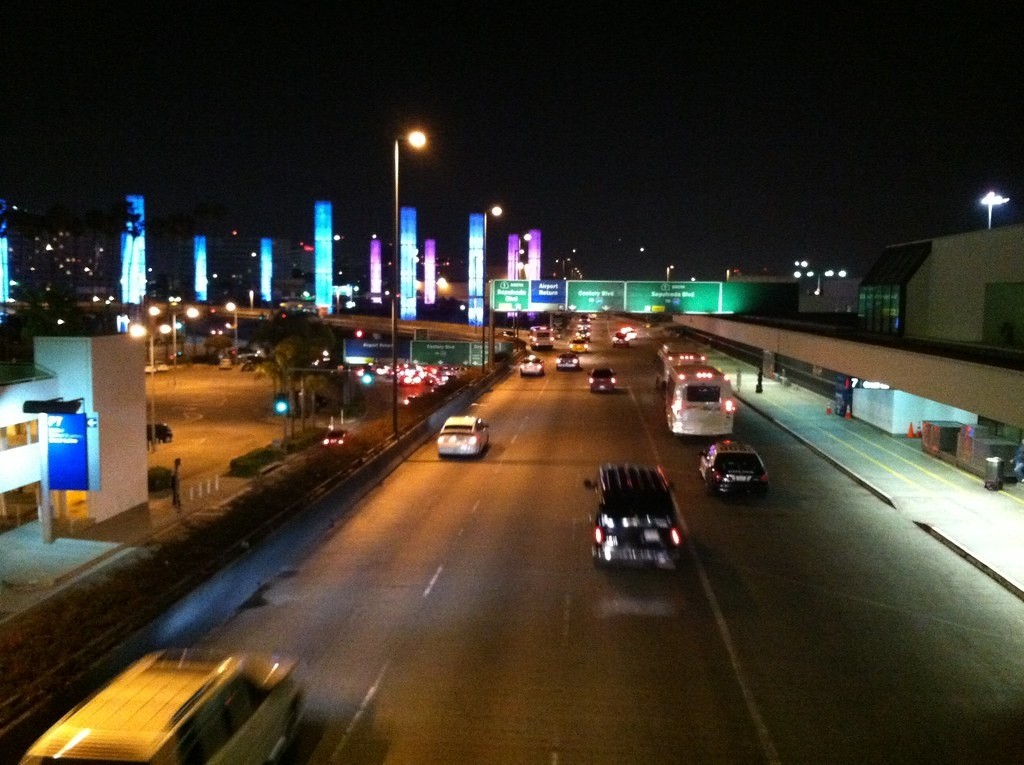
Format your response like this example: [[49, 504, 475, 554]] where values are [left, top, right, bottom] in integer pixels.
[[273, 392, 289, 416]]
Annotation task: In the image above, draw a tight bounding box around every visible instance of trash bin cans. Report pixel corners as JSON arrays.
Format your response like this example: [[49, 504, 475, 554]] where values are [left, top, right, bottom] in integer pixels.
[[984, 457, 1005, 490]]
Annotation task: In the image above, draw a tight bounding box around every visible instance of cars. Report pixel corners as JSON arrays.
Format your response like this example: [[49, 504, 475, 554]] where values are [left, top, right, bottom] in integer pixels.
[[697, 440, 770, 499], [322, 428, 353, 449], [147, 422, 172, 444], [436, 415, 490, 457], [501, 314, 639, 393]]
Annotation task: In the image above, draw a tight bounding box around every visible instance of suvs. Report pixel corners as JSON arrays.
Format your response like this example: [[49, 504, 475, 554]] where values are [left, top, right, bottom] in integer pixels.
[[584, 464, 686, 572], [17, 644, 309, 765]]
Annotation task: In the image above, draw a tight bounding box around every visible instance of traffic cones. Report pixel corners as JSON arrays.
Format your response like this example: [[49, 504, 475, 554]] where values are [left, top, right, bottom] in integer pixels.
[[844, 404, 852, 420], [906, 422, 915, 439], [824, 402, 833, 415], [915, 421, 922, 439]]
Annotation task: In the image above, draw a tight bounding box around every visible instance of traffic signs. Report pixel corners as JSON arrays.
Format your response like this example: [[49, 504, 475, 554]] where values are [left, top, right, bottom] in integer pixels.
[[564, 279, 625, 313]]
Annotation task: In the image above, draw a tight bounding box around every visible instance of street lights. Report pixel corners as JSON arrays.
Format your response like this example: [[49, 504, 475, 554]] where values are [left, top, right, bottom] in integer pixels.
[[980, 190, 1011, 231], [389, 124, 429, 440], [129, 322, 173, 453], [149, 305, 199, 387], [511, 249, 525, 344], [480, 204, 504, 375], [225, 302, 239, 358], [515, 233, 532, 355], [667, 265, 674, 281]]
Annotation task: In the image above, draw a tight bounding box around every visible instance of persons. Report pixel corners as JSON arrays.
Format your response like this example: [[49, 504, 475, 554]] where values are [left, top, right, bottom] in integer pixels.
[[839, 379, 854, 417], [1009, 439, 1024, 484]]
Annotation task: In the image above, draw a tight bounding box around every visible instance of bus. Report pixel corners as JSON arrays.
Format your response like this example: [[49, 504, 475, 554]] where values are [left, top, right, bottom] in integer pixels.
[[662, 361, 735, 438], [650, 342, 709, 395]]
